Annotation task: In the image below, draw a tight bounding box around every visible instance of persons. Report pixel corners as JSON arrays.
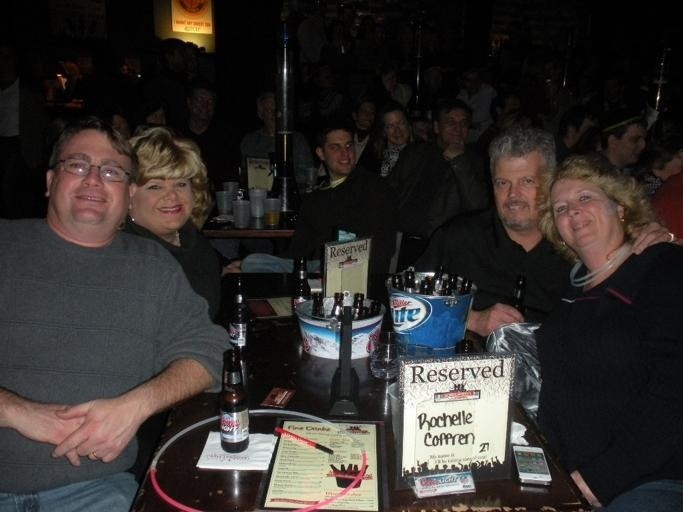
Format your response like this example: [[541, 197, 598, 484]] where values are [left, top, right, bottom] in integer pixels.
[[1, 30, 683, 339], [0, 119, 232, 512], [531, 154, 683, 511]]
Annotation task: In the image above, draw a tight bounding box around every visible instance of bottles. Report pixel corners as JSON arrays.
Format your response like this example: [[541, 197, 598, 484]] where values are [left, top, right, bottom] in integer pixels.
[[460, 337, 475, 356], [289, 254, 474, 322], [509, 274, 530, 316], [226, 275, 251, 353], [219, 349, 250, 453]]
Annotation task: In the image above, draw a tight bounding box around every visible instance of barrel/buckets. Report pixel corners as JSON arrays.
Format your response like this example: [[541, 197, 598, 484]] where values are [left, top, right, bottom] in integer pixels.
[[295, 295, 386, 362], [384, 269, 478, 350]]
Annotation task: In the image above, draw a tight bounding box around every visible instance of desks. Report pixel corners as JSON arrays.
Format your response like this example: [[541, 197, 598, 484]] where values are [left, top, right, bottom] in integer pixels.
[[201, 201, 296, 259], [128, 272, 583, 512]]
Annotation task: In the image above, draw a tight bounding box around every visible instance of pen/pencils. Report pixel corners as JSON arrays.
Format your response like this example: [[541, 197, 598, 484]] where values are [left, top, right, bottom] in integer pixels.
[[274, 426, 335, 455]]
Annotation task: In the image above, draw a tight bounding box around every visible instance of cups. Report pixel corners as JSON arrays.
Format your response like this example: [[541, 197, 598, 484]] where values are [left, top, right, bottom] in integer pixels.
[[216, 181, 281, 229], [304, 167, 318, 185], [368, 330, 434, 445]]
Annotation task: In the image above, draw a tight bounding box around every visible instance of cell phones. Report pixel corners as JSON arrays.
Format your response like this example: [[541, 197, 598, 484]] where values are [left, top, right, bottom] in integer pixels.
[[514, 444, 553, 486]]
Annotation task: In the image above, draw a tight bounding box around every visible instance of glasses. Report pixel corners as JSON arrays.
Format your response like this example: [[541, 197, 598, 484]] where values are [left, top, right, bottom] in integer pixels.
[[49, 157, 132, 183]]
